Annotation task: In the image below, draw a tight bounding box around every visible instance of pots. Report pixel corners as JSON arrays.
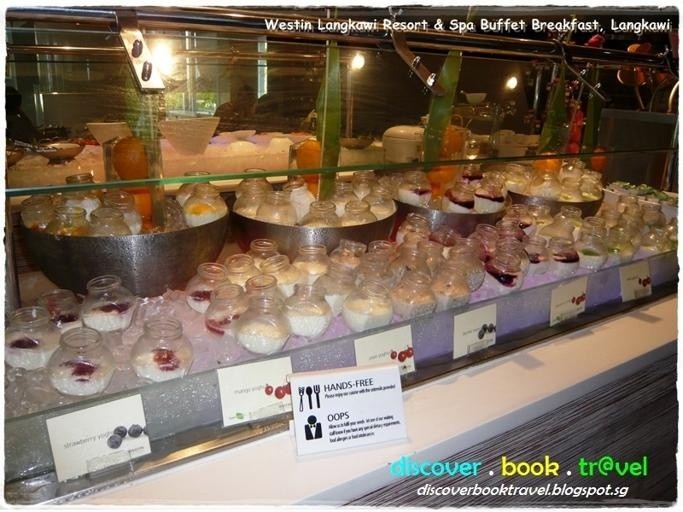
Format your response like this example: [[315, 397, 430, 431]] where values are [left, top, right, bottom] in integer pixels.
[[383, 125, 424, 164]]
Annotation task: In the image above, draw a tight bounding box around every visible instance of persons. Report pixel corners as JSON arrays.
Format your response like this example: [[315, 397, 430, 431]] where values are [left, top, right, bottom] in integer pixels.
[[212, 85, 256, 134], [5, 85, 35, 140]]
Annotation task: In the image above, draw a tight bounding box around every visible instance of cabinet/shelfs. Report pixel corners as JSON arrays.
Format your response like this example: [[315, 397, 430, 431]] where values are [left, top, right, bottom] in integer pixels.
[[6, 7, 679, 505]]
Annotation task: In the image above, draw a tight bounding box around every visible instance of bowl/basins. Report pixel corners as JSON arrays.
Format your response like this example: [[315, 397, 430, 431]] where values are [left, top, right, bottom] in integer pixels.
[[465, 93, 487, 104], [493, 129, 540, 159], [87, 122, 127, 146], [35, 142, 86, 164], [157, 117, 374, 158]]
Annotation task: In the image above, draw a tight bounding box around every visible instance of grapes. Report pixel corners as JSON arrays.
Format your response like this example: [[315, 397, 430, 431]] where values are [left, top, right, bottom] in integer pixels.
[[479, 323, 496, 339], [107, 425, 150, 448]]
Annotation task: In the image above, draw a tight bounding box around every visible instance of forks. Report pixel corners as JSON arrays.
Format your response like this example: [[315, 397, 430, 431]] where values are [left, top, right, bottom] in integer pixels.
[[298, 384, 322, 412]]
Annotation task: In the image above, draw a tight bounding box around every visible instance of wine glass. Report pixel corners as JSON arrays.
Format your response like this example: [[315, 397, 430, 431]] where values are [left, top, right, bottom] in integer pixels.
[[463, 116, 500, 181]]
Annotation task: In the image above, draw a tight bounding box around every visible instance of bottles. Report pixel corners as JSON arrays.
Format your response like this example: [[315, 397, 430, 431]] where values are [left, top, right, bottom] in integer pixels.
[[592, 146, 608, 172], [6, 167, 678, 398], [535, 152, 561, 174]]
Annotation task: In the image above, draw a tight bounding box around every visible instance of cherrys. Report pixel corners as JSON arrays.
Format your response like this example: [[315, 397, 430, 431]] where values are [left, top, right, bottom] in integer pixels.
[[266, 382, 290, 400], [639, 277, 651, 287], [571, 294, 585, 304], [390, 348, 414, 363]]
